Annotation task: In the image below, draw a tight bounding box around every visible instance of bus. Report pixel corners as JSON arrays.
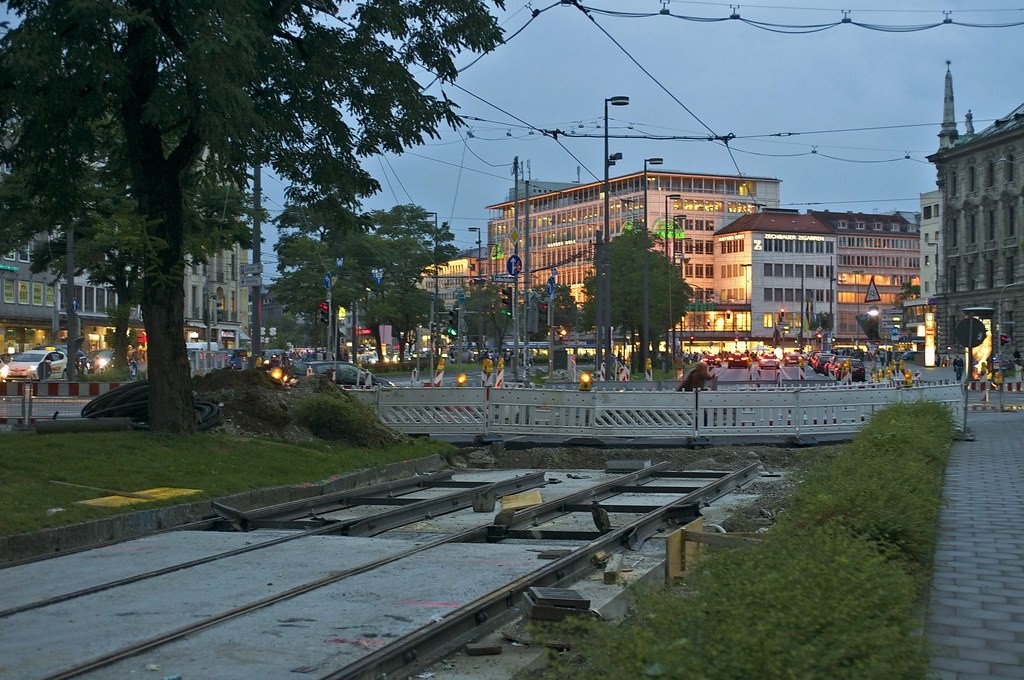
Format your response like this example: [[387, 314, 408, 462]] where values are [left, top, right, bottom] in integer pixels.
[[220, 348, 248, 369]]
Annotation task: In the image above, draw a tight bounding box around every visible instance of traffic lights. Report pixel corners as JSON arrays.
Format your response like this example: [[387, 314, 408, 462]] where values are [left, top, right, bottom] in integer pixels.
[[690, 336, 694, 340], [1001, 334, 1012, 345], [502, 287, 513, 316], [319, 303, 329, 324], [447, 309, 458, 339], [817, 334, 822, 342], [214, 300, 222, 326], [543, 301, 549, 309]]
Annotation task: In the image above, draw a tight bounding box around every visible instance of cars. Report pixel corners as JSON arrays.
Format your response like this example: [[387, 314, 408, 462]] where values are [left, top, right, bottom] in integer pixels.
[[0, 344, 87, 383], [701, 354, 719, 368], [727, 354, 749, 368], [806, 353, 865, 382], [759, 352, 779, 371], [87, 349, 138, 377], [784, 352, 802, 364]]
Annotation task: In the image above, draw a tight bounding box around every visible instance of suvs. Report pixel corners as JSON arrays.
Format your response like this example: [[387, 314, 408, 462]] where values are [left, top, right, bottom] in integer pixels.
[[901, 351, 923, 360], [283, 361, 396, 387]]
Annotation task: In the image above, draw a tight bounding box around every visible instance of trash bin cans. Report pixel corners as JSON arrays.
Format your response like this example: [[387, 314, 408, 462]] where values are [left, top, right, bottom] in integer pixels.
[[554, 350, 568, 369]]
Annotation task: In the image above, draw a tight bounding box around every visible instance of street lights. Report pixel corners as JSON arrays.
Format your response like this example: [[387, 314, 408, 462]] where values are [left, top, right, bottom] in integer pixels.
[[742, 264, 753, 353], [780, 309, 785, 317], [603, 96, 629, 382], [734, 339, 738, 342], [469, 228, 484, 357], [998, 281, 1024, 380], [489, 243, 500, 303], [422, 211, 440, 363], [664, 193, 687, 375], [852, 268, 864, 347], [643, 158, 662, 380]]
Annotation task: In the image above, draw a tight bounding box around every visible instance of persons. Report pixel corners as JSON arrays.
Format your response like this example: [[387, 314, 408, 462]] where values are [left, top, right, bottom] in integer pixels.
[[831, 347, 864, 367], [953, 353, 963, 380], [273, 349, 312, 368], [879, 349, 885, 366], [887, 348, 892, 365], [678, 362, 715, 439]]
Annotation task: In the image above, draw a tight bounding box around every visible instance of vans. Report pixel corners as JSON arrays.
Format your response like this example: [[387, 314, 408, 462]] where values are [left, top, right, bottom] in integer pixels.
[[261, 350, 289, 366], [186, 342, 219, 361]]
